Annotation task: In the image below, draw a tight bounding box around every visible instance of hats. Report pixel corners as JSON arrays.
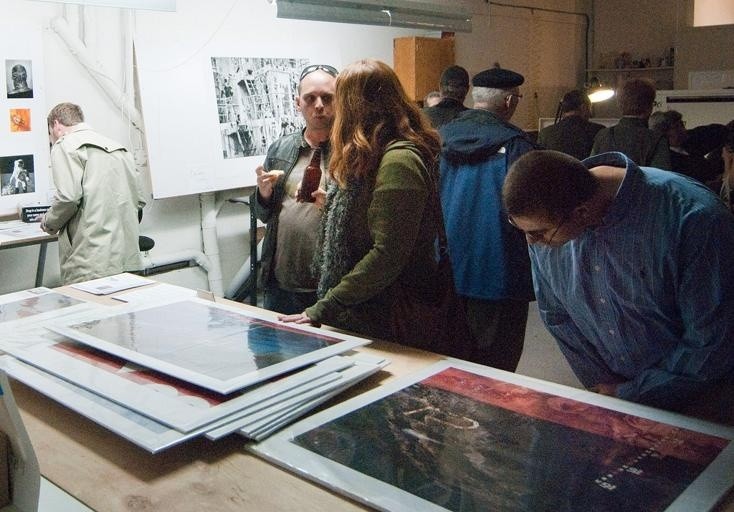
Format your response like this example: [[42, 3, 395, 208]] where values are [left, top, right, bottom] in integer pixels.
[[472, 63, 524, 89], [440, 65, 469, 88]]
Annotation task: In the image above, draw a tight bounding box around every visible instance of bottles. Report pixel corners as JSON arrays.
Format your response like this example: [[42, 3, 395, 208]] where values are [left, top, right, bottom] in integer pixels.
[[301, 149, 322, 203]]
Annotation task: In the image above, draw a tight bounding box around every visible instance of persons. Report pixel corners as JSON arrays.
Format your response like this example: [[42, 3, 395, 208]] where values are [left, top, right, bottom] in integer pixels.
[[249, 62, 340, 316], [540, 80, 733, 200], [436, 66, 536, 374], [502, 149, 734, 405], [275, 57, 453, 351], [39, 100, 148, 289], [0, 63, 35, 195], [420, 63, 473, 131]]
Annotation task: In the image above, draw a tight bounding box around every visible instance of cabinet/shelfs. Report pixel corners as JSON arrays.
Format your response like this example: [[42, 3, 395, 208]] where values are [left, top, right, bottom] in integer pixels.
[[393, 37, 455, 105]]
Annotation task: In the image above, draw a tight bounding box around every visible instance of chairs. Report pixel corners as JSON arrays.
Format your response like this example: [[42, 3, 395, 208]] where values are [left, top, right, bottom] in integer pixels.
[[139, 206, 158, 275]]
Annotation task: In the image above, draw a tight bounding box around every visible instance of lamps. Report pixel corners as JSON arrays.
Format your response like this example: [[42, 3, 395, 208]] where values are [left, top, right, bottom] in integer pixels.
[[584, 77, 613, 117]]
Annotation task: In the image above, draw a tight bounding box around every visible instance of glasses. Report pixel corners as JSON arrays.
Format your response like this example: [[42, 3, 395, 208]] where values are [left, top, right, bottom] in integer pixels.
[[503, 94, 523, 101], [507, 214, 568, 247], [300, 65, 339, 80]]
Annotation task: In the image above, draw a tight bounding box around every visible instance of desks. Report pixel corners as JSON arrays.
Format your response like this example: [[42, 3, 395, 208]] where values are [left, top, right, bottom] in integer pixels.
[[0, 274, 731, 512], [0, 219, 59, 288]]
